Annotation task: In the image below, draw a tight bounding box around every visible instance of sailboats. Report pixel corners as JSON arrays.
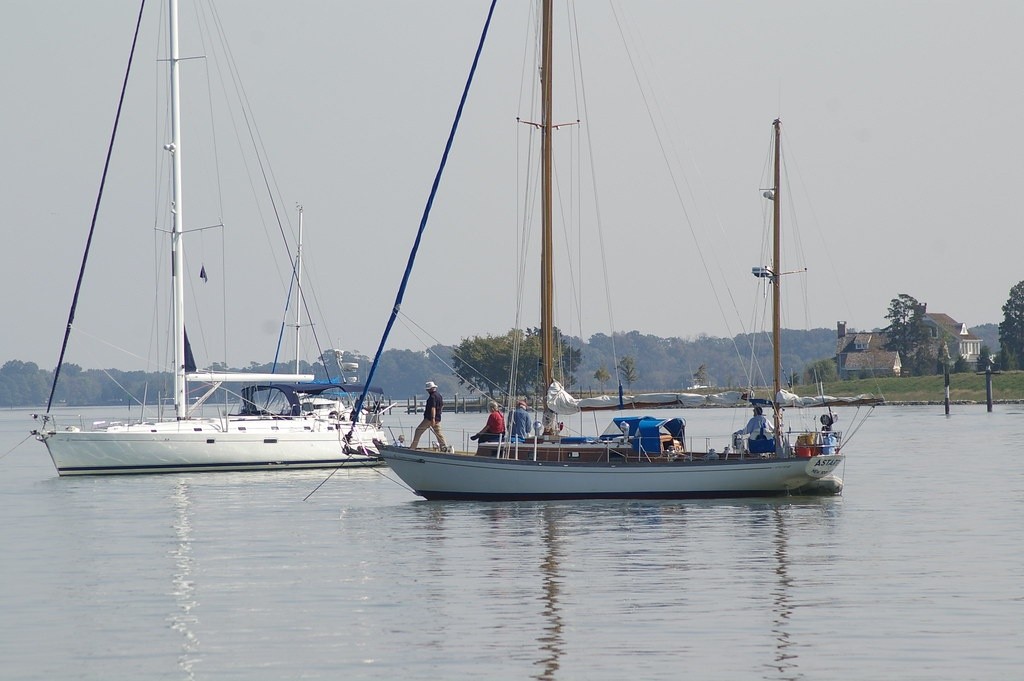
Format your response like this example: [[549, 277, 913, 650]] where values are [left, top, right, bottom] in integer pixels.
[[31, 1, 408, 478], [329, 0, 887, 500]]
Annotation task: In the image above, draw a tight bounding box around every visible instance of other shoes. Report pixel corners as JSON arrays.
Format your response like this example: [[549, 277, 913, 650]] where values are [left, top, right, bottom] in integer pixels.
[[470, 433, 481, 441]]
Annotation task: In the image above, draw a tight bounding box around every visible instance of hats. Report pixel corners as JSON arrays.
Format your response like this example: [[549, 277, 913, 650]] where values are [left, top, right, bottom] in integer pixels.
[[423, 382, 438, 390], [516, 401, 528, 406]]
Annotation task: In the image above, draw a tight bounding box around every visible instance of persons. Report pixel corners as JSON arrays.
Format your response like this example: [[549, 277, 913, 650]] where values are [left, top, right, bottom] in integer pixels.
[[350, 395, 368, 422], [734, 407, 795, 454], [470, 401, 505, 442], [392, 435, 404, 447], [507, 401, 531, 442], [408, 381, 447, 452]]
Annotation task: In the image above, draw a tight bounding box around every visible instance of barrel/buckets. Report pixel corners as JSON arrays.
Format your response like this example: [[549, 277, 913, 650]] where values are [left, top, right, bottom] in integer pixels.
[[795, 434, 821, 457]]
